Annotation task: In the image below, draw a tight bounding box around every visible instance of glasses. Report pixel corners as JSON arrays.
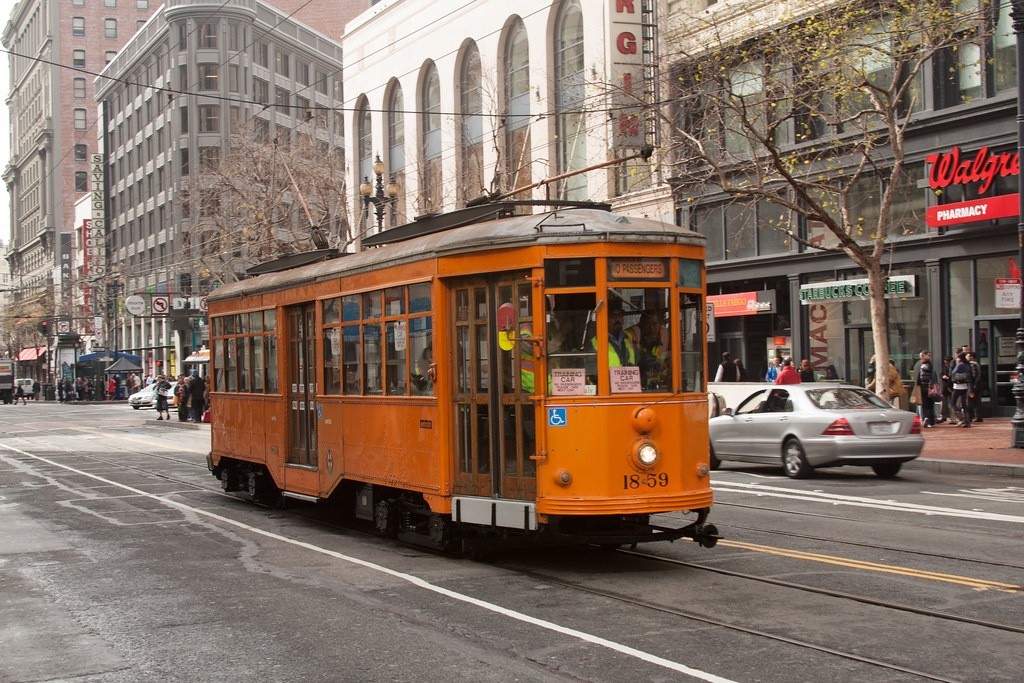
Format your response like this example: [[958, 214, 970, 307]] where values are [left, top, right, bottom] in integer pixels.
[[609, 311, 624, 320]]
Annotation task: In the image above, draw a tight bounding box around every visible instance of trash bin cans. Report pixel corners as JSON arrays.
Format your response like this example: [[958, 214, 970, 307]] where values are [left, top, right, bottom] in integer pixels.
[[46, 386, 56, 401]]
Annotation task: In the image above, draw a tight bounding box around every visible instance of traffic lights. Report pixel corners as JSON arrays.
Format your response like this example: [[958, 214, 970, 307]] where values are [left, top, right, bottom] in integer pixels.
[[42, 321, 47, 333]]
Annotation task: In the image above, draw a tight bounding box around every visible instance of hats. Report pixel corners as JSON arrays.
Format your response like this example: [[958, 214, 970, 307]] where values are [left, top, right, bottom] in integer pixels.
[[958, 352, 966, 358]]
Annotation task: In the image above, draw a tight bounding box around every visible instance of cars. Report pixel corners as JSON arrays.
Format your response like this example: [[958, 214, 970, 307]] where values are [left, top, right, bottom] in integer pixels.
[[708, 383, 923, 478], [130, 381, 180, 410]]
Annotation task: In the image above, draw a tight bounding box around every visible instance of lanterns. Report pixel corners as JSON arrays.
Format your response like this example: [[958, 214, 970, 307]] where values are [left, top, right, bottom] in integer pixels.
[[157, 362, 160, 367]]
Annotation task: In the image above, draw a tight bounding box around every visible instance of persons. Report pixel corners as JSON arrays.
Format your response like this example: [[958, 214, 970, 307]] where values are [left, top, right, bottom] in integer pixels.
[[520, 296, 672, 477], [14, 384, 26, 405], [411, 341, 432, 393], [144, 370, 210, 423], [864, 345, 982, 428], [766, 357, 838, 385], [32, 372, 143, 403], [715, 353, 747, 381]]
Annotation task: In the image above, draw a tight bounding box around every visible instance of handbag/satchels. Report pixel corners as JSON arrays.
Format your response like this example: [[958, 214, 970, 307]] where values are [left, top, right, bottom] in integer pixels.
[[952, 372, 969, 384], [928, 383, 944, 401], [909, 385, 922, 405], [158, 388, 167, 396]]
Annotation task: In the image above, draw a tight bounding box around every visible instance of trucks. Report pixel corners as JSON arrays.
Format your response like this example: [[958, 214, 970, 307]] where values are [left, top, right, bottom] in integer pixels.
[[0, 358, 14, 405]]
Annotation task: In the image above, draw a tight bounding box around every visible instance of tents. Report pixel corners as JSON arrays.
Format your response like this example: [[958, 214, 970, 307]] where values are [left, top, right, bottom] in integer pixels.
[[78, 351, 142, 361], [104, 356, 143, 394]]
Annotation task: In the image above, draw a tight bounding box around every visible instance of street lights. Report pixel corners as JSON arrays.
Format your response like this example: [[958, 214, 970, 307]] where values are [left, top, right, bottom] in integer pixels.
[[104, 265, 125, 361], [358, 156, 402, 233]]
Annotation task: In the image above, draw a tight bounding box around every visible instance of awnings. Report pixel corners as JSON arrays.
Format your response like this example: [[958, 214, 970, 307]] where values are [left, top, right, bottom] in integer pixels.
[[182, 349, 210, 364]]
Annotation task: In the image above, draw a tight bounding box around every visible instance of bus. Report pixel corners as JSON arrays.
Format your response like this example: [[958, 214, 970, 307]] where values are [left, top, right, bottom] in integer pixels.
[[205, 200, 725, 556]]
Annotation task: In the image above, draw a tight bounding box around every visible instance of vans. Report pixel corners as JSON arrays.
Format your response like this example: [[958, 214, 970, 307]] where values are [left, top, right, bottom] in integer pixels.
[[14, 378, 34, 400]]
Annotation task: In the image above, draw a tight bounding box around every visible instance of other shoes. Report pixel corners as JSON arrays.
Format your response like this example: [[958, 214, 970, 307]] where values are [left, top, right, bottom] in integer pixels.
[[167, 415, 170, 420], [923, 417, 932, 428], [956, 422, 971, 428], [970, 419, 983, 424], [157, 416, 163, 420], [949, 422, 957, 424], [941, 418, 946, 421]]
